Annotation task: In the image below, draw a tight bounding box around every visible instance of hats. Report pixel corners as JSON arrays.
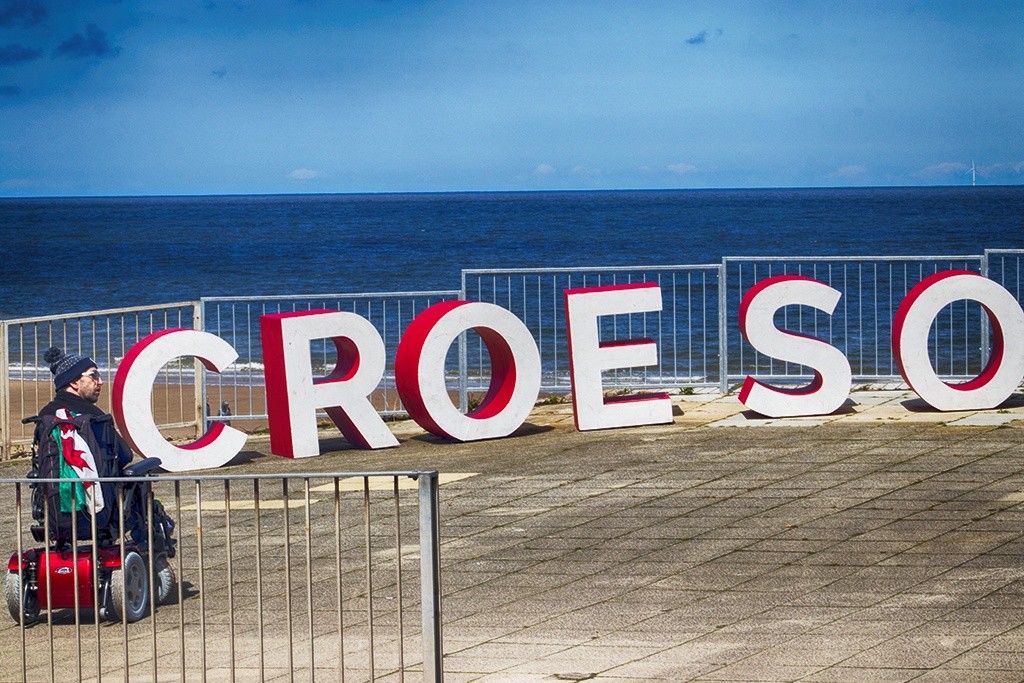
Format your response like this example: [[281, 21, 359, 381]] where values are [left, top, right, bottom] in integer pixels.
[[44, 346, 99, 392]]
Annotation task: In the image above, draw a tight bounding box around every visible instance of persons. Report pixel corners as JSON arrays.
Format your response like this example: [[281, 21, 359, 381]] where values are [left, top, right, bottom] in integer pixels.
[[33, 346, 175, 541], [206, 395, 211, 431], [217, 399, 232, 425]]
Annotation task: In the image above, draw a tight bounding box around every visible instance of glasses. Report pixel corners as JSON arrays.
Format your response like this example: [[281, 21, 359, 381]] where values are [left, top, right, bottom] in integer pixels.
[[82, 371, 100, 379]]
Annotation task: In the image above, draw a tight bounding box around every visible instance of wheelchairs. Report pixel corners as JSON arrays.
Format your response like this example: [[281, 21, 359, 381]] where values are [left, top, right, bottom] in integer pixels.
[[4, 414, 176, 624]]
